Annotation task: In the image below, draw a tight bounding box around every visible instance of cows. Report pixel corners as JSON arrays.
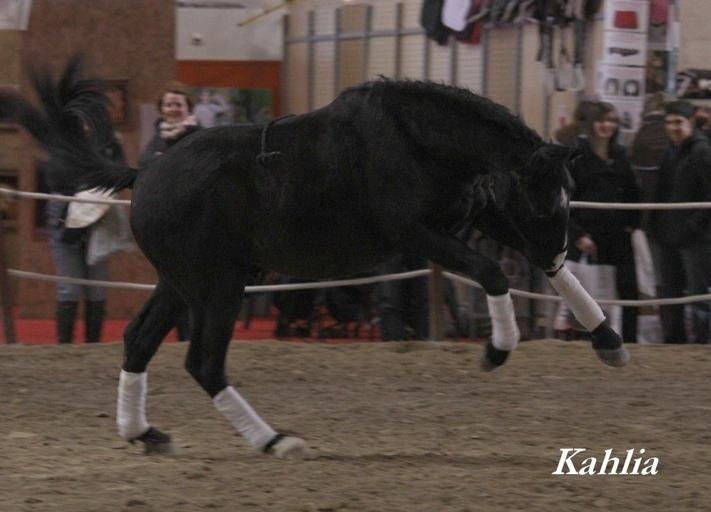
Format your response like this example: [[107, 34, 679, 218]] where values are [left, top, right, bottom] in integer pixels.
[[19, 49, 632, 461]]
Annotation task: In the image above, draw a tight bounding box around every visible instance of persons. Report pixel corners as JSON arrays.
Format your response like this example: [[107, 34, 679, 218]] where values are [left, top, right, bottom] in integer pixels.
[[137, 81, 210, 340], [46, 114, 132, 344], [193, 87, 229, 127]]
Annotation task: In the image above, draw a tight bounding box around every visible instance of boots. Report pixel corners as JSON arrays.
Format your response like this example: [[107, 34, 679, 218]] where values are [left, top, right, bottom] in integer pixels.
[[84, 300, 105, 343], [56, 300, 79, 344]]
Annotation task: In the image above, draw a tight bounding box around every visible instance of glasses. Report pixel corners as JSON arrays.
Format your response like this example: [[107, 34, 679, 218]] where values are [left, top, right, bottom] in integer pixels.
[[595, 116, 618, 122], [663, 118, 684, 124]]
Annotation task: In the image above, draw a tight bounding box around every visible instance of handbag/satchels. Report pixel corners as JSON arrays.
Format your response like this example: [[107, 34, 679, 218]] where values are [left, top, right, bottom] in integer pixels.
[[554, 248, 623, 335]]
